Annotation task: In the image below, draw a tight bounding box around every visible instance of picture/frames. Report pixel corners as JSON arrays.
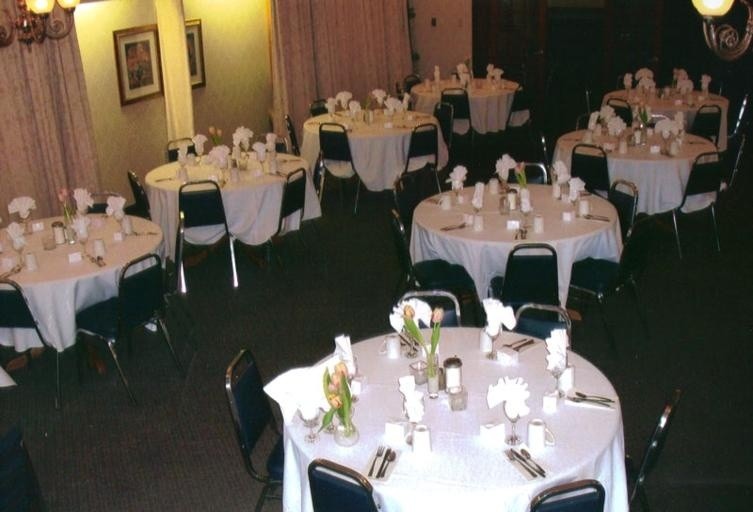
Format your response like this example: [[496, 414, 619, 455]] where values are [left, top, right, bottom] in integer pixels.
[[114, 24, 164, 107], [185, 19, 206, 89]]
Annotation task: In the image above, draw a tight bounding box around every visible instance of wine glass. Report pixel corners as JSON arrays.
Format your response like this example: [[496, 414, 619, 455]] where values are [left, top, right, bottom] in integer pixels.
[[170, 139, 284, 186], [502, 394, 525, 448], [17, 214, 35, 247], [470, 196, 484, 233], [404, 323, 419, 358], [624, 78, 712, 109], [326, 98, 421, 129], [401, 388, 424, 444], [544, 342, 567, 400], [481, 315, 503, 362], [111, 207, 125, 233], [556, 208, 574, 238], [582, 113, 688, 157], [75, 227, 93, 263], [10, 236, 29, 269], [421, 71, 503, 94], [295, 354, 358, 445], [518, 187, 534, 230], [566, 179, 583, 219], [550, 164, 569, 203]]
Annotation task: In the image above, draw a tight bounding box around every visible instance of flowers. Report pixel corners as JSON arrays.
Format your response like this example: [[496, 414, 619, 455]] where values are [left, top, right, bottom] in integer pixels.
[[59, 188, 73, 225], [399, 305, 443, 377], [209, 127, 223, 147], [317, 364, 359, 437]]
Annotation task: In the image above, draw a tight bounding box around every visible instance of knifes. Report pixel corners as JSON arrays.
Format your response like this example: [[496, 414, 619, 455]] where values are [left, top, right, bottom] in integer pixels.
[[501, 337, 537, 355], [82, 253, 110, 269], [583, 211, 616, 225], [1, 263, 22, 283], [376, 446, 390, 480]]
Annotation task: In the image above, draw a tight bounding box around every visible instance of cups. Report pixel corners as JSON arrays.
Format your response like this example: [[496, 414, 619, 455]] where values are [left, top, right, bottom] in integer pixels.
[[526, 416, 556, 452], [408, 359, 429, 386], [23, 248, 38, 272], [558, 363, 576, 392], [449, 177, 464, 211], [377, 333, 404, 360], [532, 212, 548, 235], [576, 198, 593, 222], [484, 177, 500, 195], [409, 425, 438, 460], [496, 195, 512, 219], [448, 385, 467, 411], [436, 193, 453, 212]]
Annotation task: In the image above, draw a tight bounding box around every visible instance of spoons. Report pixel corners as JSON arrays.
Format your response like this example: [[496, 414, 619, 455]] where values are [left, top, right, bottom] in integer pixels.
[[437, 217, 468, 234], [381, 451, 396, 479], [566, 391, 615, 410]]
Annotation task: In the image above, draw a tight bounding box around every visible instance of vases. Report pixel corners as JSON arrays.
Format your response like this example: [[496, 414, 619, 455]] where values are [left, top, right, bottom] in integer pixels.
[[66, 221, 78, 246], [334, 407, 361, 447], [426, 355, 439, 400]]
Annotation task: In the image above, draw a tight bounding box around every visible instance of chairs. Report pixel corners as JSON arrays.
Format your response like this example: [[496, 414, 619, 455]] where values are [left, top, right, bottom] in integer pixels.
[[529, 480, 606, 511], [86, 135, 307, 292], [285, 68, 557, 213], [390, 163, 655, 358], [1, 275, 69, 414], [224, 347, 287, 512], [628, 387, 684, 511], [73, 254, 183, 405], [533, 76, 750, 260], [306, 457, 384, 511]]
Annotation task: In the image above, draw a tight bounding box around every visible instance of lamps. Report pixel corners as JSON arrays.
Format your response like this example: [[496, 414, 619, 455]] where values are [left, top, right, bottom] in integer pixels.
[[0, 0, 80, 53], [692, 0, 753, 61]]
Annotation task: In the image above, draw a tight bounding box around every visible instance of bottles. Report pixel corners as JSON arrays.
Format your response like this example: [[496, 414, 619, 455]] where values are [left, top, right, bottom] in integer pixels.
[[436, 367, 446, 391], [51, 219, 67, 246], [443, 355, 464, 396]]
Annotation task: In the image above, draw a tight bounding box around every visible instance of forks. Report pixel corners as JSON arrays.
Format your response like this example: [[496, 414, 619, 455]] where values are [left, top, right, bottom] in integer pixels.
[[367, 444, 385, 479], [502, 448, 547, 482]]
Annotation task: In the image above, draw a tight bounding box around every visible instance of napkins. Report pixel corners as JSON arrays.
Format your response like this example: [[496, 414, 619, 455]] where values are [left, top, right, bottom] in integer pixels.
[[298, 378, 320, 421], [675, 71, 688, 84], [495, 154, 518, 180], [233, 127, 253, 151], [635, 69, 654, 82], [398, 375, 425, 422], [624, 73, 633, 88], [6, 222, 29, 250], [74, 188, 94, 212], [487, 374, 531, 418], [333, 334, 356, 375], [545, 328, 569, 370], [481, 423, 508, 456], [483, 297, 518, 339], [389, 300, 432, 334], [73, 217, 91, 239], [7, 197, 38, 219], [382, 423, 407, 451]]
